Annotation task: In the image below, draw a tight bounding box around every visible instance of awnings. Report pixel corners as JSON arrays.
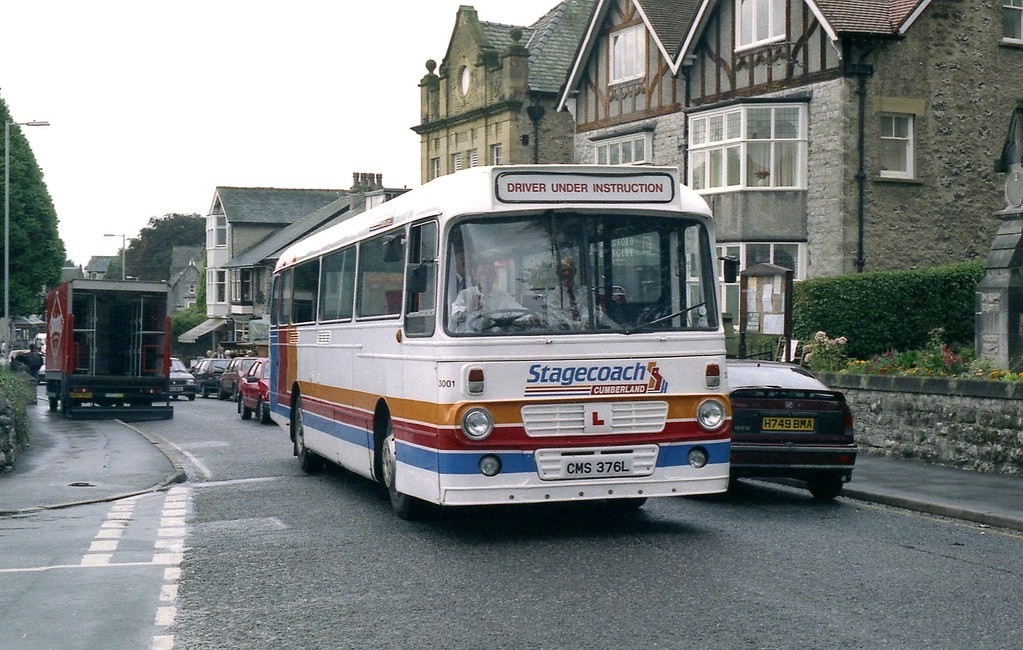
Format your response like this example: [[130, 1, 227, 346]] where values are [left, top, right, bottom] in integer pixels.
[[177, 316, 234, 343]]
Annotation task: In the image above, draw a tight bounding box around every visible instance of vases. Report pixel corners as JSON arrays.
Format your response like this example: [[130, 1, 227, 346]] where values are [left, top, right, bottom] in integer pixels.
[[758, 179, 766, 187]]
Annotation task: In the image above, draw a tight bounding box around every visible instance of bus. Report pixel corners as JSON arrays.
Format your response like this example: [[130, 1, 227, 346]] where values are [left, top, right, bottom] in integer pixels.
[[260, 162, 733, 522]]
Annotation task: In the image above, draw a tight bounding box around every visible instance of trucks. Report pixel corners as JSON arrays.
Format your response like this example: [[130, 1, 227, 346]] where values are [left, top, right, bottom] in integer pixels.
[[47, 278, 177, 421]]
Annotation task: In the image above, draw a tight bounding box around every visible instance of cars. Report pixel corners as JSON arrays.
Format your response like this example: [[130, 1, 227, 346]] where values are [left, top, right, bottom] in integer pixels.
[[239, 358, 274, 425], [722, 357, 860, 496], [218, 357, 265, 403], [11, 333, 48, 384], [167, 357, 197, 401], [189, 358, 231, 398]]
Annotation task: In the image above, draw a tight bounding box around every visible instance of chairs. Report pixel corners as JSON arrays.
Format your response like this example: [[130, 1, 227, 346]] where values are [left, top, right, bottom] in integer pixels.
[[594, 285, 628, 329]]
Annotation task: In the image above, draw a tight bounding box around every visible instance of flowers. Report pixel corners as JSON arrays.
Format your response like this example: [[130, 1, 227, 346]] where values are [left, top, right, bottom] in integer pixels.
[[754, 170, 771, 179]]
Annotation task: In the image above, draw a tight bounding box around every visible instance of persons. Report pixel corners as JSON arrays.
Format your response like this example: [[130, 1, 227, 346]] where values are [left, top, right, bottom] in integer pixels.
[[546, 258, 622, 331], [206, 346, 258, 358], [449, 259, 543, 334], [24, 343, 43, 405]]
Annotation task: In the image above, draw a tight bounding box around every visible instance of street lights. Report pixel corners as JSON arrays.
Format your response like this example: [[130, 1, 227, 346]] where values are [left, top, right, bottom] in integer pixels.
[[104, 233, 130, 282], [4, 120, 49, 352]]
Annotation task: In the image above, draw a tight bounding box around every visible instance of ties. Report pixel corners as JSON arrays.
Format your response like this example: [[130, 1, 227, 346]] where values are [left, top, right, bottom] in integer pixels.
[[483, 295, 492, 333], [567, 288, 581, 321]]
